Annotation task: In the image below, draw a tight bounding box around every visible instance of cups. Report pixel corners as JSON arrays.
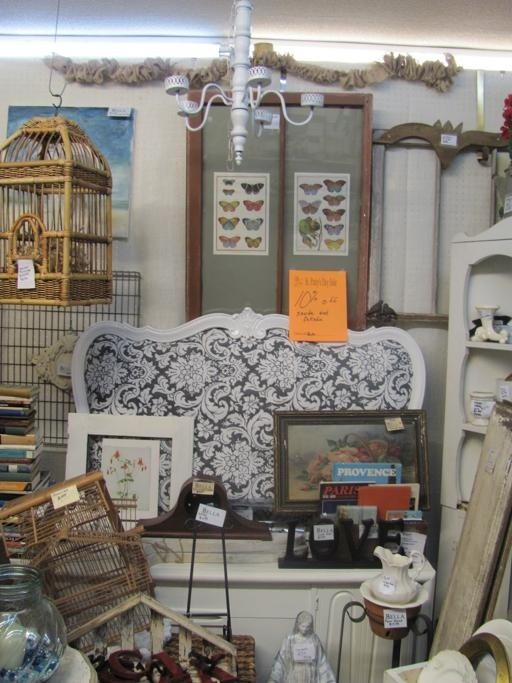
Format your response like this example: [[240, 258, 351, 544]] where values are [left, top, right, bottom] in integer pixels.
[[468, 391, 494, 426]]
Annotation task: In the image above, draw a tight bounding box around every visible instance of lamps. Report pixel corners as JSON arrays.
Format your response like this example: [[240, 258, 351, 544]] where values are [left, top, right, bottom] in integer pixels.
[[161, 1, 325, 169]]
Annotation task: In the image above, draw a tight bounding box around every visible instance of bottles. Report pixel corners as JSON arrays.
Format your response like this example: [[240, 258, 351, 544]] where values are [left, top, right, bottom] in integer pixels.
[[0, 565, 66, 683]]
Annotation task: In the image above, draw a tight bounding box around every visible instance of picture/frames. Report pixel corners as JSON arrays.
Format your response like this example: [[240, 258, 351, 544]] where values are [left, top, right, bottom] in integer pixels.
[[65, 412, 194, 532], [272, 409, 432, 520]]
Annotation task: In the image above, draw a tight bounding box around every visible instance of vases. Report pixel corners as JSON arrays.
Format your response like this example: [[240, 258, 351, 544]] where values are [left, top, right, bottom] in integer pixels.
[[363, 598, 422, 640], [370, 544, 417, 604]]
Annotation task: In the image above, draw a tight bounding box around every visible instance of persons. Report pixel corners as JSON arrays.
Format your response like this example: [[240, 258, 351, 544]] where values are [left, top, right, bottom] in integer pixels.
[[267, 611, 338, 683]]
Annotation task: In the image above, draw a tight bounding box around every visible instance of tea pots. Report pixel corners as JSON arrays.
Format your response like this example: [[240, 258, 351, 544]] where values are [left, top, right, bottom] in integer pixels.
[[370, 547, 426, 604]]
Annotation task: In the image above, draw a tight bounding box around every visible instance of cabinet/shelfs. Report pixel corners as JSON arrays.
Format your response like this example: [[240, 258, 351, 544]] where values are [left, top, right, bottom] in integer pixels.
[[432, 215, 512, 628], [55, 531, 435, 683]]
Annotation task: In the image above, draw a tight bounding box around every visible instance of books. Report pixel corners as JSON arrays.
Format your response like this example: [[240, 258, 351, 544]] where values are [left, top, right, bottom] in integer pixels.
[[318, 460, 421, 525], [0, 381, 54, 509]]
